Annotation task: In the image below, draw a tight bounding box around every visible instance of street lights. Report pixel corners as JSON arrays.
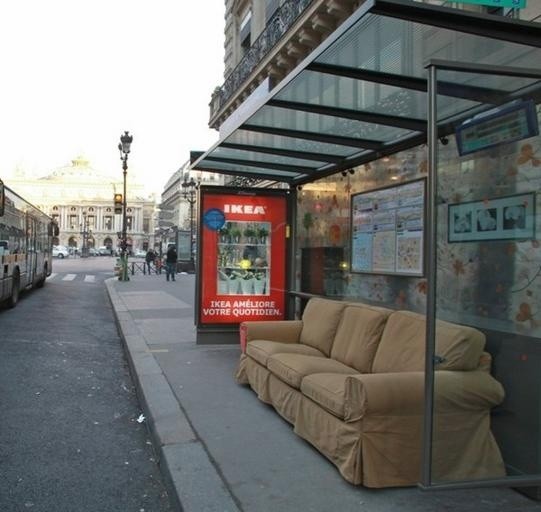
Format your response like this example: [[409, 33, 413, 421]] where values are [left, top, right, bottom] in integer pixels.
[[182, 177, 201, 275], [116, 130, 134, 282]]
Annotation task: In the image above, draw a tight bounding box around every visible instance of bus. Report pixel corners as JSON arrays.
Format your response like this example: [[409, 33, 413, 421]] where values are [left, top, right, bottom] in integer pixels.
[[0, 181, 59, 314]]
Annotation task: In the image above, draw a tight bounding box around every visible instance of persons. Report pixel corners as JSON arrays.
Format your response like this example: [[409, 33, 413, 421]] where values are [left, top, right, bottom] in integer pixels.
[[497, 142, 541, 183], [145, 249, 159, 264], [166, 245, 178, 281]]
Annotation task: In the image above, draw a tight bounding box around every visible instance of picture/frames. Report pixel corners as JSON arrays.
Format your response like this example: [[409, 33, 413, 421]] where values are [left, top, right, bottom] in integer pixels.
[[348, 177, 428, 279], [447, 190, 536, 244]]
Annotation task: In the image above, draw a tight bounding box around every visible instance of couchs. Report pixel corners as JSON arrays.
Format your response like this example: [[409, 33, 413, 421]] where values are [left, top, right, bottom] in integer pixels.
[[236, 297, 506, 490]]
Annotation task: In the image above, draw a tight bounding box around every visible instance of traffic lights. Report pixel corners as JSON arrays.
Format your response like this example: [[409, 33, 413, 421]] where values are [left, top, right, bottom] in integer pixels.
[[114, 193, 122, 214]]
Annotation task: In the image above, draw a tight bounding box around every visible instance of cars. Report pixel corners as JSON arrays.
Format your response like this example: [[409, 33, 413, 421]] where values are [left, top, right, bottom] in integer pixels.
[[51, 245, 148, 260]]
